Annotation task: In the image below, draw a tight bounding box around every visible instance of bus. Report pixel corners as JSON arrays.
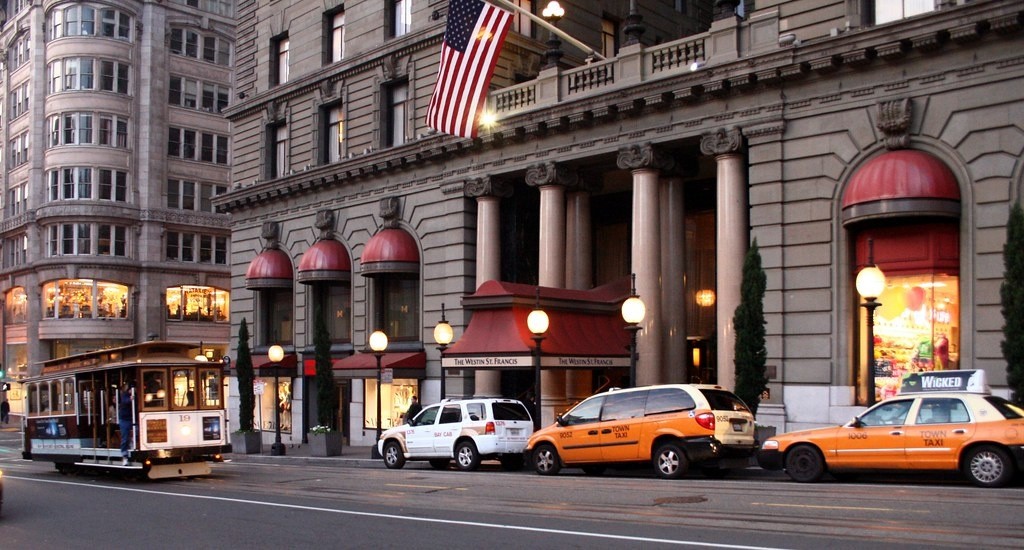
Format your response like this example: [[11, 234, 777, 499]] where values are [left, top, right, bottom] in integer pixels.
[[16, 339, 234, 480]]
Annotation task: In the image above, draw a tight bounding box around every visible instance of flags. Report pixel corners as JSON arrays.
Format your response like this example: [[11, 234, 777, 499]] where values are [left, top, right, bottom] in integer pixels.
[[424, 0, 514, 137]]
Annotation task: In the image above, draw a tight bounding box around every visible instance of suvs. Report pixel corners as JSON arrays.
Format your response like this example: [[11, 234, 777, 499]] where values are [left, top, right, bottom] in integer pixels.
[[525, 384, 756, 479], [376, 396, 535, 469]]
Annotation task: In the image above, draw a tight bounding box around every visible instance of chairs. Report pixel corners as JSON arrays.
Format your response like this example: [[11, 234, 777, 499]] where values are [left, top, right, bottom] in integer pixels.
[[932, 407, 951, 423]]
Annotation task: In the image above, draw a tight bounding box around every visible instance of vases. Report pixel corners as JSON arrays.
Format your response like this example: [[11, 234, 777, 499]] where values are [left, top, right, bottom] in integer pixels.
[[723, 236, 778, 468]]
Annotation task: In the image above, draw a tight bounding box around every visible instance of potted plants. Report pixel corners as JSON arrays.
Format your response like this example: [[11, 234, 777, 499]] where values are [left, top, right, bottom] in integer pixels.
[[229, 316, 261, 454], [307, 303, 344, 457]]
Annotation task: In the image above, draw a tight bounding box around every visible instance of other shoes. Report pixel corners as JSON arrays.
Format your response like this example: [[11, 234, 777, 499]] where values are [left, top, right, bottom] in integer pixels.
[[122, 458, 129, 466]]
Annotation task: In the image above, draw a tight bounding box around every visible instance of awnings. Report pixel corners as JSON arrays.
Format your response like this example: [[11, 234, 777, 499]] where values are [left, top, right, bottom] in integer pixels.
[[226, 351, 424, 378]]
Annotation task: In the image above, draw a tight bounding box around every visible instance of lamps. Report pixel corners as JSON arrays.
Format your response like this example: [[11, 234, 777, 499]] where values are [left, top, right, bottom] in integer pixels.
[[431, 11, 447, 21], [239, 92, 250, 100]]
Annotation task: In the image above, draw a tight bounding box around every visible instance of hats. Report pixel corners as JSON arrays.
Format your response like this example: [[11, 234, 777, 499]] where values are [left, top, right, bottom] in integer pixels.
[[127, 381, 136, 392]]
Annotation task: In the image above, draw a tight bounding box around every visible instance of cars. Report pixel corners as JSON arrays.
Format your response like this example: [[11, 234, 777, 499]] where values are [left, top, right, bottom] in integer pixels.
[[757, 369, 1024, 490]]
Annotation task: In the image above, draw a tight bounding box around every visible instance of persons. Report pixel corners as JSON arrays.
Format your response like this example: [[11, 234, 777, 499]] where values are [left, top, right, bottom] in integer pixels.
[[408, 396, 422, 422], [0, 399, 11, 424], [42, 377, 194, 468], [524, 393, 537, 421]]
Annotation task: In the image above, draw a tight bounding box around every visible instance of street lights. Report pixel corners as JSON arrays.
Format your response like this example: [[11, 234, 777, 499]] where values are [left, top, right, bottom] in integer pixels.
[[267, 329, 287, 456], [433, 303, 454, 403], [369, 305, 389, 459], [621, 272, 646, 388], [855, 238, 886, 408], [194, 341, 208, 405], [526, 286, 550, 433]]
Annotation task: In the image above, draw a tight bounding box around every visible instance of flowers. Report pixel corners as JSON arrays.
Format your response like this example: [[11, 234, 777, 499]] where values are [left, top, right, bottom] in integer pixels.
[[309, 424, 337, 436]]
